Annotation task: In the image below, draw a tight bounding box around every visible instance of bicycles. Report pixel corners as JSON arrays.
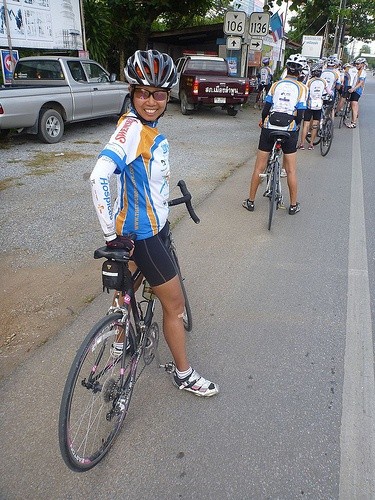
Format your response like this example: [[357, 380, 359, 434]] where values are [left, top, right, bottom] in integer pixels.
[[259, 130, 291, 231], [307, 95, 334, 157], [258, 81, 267, 109], [57, 180, 200, 473], [339, 92, 352, 129]]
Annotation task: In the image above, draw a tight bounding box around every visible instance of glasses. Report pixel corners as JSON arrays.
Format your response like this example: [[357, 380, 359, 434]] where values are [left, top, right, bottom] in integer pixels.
[[134, 88, 168, 101]]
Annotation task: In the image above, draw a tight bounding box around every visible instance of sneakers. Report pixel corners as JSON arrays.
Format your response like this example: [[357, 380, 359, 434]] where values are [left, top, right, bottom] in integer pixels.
[[110, 343, 131, 359], [173, 369, 218, 397]]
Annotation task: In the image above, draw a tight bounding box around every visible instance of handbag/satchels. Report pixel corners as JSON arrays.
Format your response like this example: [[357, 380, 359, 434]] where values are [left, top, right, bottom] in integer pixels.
[[268, 111, 294, 126]]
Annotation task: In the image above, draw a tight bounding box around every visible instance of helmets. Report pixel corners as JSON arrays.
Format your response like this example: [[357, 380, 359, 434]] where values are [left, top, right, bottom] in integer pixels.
[[123, 49, 178, 90], [262, 57, 269, 64], [286, 54, 369, 77]]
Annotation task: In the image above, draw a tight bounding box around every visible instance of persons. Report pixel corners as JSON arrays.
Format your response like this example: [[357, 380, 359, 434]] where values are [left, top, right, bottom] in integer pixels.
[[89, 50, 220, 397], [242, 53, 307, 215], [253, 55, 367, 150]]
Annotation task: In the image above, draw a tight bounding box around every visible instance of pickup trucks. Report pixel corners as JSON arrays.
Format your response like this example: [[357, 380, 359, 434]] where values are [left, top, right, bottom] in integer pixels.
[[168, 53, 250, 116], [0, 55, 133, 145]]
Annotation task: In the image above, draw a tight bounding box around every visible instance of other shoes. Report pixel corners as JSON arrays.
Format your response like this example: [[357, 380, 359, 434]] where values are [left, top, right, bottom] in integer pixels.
[[308, 145, 315, 150], [242, 199, 254, 211], [289, 201, 301, 215], [347, 122, 356, 128], [297, 144, 304, 149]]
